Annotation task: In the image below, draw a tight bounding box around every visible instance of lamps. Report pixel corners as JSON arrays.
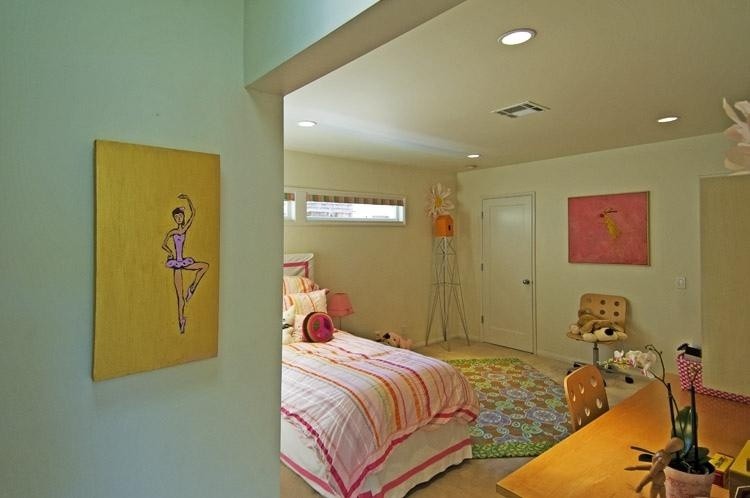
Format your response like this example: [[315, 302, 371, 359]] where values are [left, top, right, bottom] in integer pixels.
[[328, 293, 354, 330]]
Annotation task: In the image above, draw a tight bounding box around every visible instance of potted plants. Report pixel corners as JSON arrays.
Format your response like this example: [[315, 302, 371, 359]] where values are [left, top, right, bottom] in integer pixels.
[[661, 406, 716, 498]]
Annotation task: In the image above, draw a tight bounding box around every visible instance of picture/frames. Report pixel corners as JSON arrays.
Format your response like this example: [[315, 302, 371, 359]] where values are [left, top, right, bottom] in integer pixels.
[[567, 191, 651, 265]]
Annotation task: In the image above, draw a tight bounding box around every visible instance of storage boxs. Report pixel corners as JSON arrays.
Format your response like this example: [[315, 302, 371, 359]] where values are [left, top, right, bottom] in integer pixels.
[[707, 440, 749, 497]]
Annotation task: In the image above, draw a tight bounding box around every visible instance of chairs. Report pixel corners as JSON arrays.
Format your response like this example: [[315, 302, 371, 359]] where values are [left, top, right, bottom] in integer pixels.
[[564, 365, 610, 433], [567, 294, 635, 386]]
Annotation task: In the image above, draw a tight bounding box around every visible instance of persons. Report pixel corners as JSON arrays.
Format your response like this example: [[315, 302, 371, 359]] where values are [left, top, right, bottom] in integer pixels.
[[160, 194, 210, 336], [624, 437, 684, 498]]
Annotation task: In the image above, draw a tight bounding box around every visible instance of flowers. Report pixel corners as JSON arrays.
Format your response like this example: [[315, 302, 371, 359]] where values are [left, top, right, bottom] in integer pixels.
[[425, 184, 457, 224]]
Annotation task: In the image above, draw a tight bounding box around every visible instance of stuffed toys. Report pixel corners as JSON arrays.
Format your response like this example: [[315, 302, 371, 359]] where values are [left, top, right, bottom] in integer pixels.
[[375, 331, 412, 350], [569, 310, 628, 342]]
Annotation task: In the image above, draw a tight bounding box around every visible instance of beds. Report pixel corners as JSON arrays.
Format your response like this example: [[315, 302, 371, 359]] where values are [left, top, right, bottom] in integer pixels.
[[282, 252, 455, 498]]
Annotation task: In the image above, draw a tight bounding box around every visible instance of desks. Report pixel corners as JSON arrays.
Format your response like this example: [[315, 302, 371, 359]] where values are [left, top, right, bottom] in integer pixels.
[[495, 371, 750, 497]]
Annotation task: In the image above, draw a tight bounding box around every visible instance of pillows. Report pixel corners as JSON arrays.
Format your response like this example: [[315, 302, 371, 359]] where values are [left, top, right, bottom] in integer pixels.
[[283, 276, 333, 344]]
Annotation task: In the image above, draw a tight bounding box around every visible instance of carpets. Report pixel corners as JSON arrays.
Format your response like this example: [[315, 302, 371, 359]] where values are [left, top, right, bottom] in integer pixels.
[[429, 358, 572, 459]]
